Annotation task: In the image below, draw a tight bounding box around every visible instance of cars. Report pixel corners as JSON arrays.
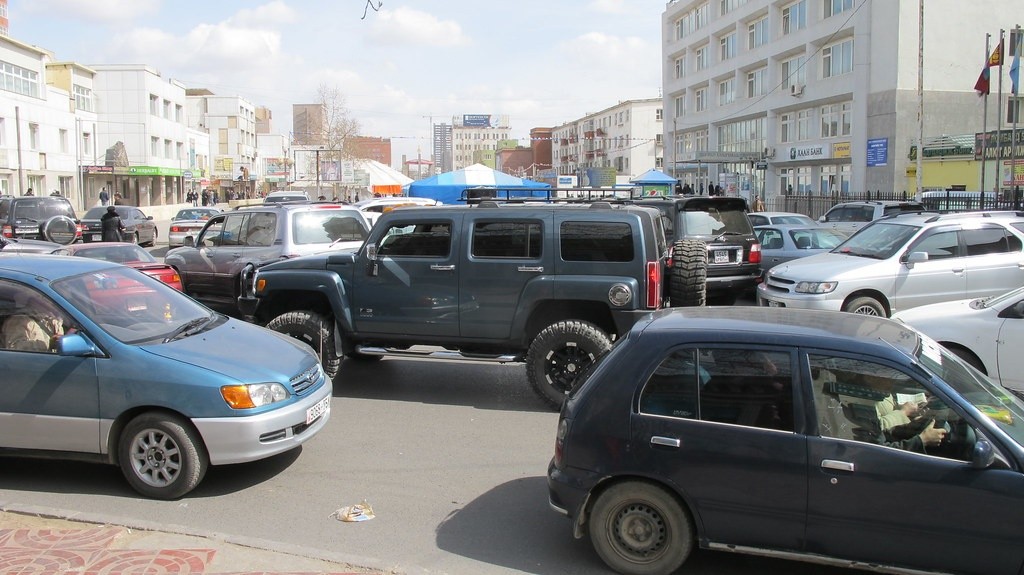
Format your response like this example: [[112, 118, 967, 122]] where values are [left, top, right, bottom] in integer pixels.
[[353, 195, 444, 247], [168, 207, 224, 249], [81, 205, 159, 247], [545, 304, 1024, 575], [889, 286, 1024, 393], [48, 242, 184, 323], [0, 250, 332, 502], [746, 201, 928, 273]]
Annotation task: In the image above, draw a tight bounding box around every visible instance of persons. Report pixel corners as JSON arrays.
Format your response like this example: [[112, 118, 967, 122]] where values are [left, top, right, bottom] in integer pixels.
[[700, 183, 703, 195], [114, 191, 123, 206], [100, 187, 109, 206], [701, 353, 777, 428], [582, 171, 589, 186], [327, 161, 337, 179], [675, 183, 694, 195], [240, 167, 248, 179], [317, 192, 397, 202], [840, 374, 946, 455], [709, 181, 722, 196], [101, 205, 124, 242], [187, 189, 269, 207], [24, 188, 34, 196], [0, 282, 77, 353], [752, 195, 764, 212]]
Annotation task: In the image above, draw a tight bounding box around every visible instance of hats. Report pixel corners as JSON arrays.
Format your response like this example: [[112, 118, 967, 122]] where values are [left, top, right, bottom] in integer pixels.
[[107, 206, 115, 210]]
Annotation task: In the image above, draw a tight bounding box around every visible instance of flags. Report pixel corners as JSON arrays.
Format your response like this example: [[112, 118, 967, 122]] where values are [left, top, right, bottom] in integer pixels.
[[974, 38, 1004, 97], [1009, 35, 1022, 94]]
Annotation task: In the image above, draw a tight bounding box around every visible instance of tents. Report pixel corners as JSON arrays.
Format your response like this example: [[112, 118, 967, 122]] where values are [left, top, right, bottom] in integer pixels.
[[291, 158, 413, 202], [408, 162, 551, 205]]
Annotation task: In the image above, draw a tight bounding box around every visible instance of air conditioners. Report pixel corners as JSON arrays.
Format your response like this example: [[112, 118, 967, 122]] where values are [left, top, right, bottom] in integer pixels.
[[789, 84, 802, 95], [764, 147, 772, 157]]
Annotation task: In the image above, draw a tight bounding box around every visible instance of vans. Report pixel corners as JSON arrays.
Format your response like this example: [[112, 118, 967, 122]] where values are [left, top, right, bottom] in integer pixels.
[[911, 190, 1004, 214]]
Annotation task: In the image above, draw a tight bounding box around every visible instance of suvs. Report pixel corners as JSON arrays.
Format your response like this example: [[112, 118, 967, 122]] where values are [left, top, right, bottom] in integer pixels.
[[608, 195, 765, 304], [237, 200, 709, 414], [262, 189, 311, 203], [755, 208, 1024, 320], [0, 194, 83, 245], [164, 200, 373, 312]]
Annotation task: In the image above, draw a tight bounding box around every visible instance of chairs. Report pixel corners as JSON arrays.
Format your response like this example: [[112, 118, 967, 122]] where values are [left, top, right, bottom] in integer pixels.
[[769, 237, 783, 249], [797, 237, 811, 247]]
